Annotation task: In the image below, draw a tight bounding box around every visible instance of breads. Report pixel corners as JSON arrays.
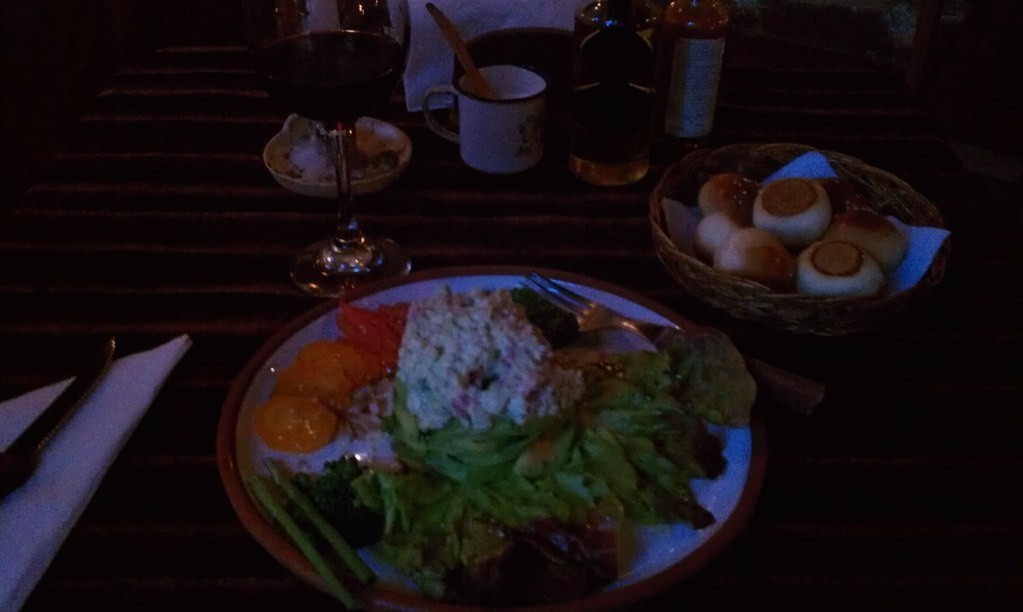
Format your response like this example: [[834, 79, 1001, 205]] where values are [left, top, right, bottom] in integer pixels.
[[696, 174, 905, 294]]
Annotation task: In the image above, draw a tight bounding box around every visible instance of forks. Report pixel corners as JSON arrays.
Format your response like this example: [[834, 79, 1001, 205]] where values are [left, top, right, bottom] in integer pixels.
[[512, 271, 827, 418]]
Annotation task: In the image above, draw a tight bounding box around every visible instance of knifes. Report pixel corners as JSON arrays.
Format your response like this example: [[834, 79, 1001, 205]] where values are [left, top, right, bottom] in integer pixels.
[[0, 336, 117, 502]]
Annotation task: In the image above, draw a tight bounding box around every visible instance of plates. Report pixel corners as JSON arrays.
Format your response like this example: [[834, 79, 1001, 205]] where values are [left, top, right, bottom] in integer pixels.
[[455, 27, 574, 138], [216, 265, 769, 611], [262, 111, 413, 196]]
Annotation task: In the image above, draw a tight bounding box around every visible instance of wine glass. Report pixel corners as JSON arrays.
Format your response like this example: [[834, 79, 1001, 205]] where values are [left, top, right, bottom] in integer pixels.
[[244, 0, 413, 301]]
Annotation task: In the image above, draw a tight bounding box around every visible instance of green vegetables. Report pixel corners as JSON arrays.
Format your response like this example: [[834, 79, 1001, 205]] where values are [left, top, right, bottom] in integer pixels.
[[350, 334, 756, 580]]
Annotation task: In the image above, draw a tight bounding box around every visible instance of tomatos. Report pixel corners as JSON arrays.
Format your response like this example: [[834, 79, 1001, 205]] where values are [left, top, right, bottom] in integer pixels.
[[251, 297, 408, 452]]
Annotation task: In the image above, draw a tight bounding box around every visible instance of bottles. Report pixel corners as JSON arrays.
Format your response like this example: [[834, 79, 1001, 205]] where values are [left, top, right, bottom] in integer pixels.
[[652, 0, 729, 140], [567, 0, 656, 187]]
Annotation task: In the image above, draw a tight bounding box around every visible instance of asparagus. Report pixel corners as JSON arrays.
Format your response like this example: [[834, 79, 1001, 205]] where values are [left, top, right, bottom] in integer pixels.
[[247, 456, 376, 607]]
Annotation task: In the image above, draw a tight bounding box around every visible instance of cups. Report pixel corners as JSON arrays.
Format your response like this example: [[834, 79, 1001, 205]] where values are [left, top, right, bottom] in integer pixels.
[[423, 66, 548, 174]]
